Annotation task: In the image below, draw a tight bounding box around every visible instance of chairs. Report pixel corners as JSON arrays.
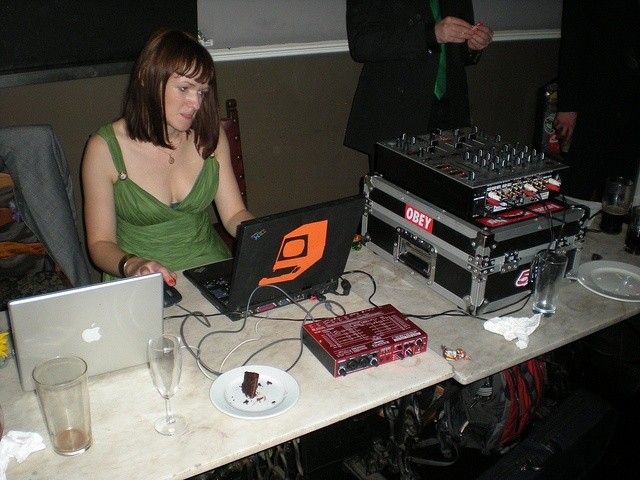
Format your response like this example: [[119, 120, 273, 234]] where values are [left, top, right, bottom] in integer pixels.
[[1, 127, 71, 290], [211, 99, 247, 261]]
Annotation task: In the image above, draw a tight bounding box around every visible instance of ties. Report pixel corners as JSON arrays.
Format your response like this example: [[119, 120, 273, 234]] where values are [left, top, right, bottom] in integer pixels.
[[428, 0, 447, 101]]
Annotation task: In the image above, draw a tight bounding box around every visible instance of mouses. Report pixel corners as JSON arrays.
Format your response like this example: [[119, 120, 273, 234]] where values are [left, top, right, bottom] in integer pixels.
[[163, 282, 183, 307]]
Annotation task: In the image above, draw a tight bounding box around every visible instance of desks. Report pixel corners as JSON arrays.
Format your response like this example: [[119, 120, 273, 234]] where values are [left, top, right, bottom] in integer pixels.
[[1, 257, 454, 476], [338, 176, 640, 385]]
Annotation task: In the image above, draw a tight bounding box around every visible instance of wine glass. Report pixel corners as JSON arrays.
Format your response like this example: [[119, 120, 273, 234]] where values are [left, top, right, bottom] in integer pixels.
[[146, 335, 189, 438]]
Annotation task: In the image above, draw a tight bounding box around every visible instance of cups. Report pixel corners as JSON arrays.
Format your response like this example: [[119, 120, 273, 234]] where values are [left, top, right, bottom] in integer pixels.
[[531, 251, 569, 317], [31, 356, 93, 457], [600, 175, 633, 234]]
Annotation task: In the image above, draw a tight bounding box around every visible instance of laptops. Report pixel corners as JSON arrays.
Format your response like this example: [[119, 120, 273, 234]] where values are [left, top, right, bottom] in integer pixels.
[[7, 272, 164, 392], [183, 194, 367, 322]]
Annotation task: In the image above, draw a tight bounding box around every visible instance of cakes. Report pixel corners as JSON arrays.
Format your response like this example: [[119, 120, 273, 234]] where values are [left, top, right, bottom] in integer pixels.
[[241, 371, 259, 399]]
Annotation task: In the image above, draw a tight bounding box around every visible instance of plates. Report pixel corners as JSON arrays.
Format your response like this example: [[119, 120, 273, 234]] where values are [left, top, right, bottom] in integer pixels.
[[209, 364, 300, 420], [576, 259, 639, 302]]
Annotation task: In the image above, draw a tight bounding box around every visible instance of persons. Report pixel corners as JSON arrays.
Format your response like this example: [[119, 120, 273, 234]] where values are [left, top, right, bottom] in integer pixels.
[[552, 0, 640, 203], [80, 28, 259, 286], [343, 0, 494, 175]]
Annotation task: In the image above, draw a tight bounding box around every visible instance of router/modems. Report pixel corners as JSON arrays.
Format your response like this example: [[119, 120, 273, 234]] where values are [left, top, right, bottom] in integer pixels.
[[301, 304, 429, 378]]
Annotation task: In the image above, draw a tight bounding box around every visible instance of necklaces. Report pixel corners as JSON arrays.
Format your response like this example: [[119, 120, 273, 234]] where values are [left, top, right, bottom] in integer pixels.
[[153, 131, 183, 164]]
